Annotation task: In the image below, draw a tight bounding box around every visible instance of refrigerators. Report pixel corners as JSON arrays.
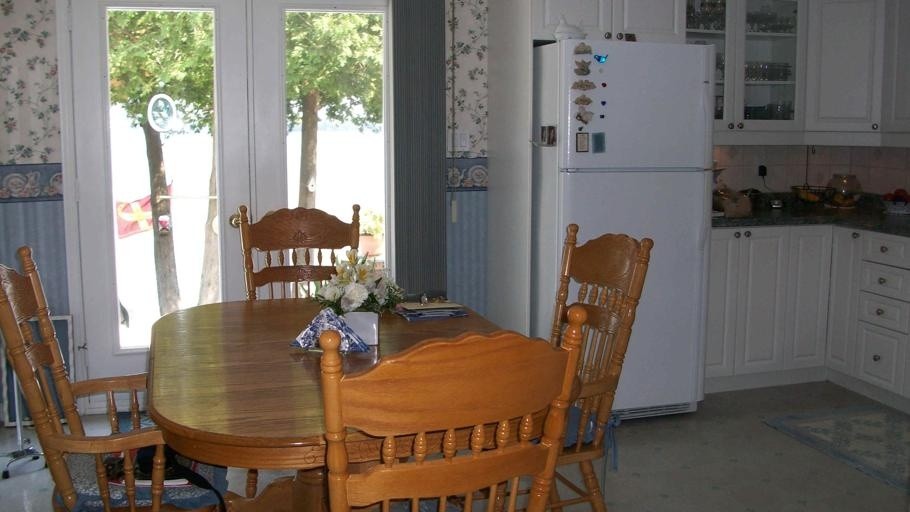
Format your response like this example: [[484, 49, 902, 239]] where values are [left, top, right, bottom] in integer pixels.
[[533, 35, 718, 424]]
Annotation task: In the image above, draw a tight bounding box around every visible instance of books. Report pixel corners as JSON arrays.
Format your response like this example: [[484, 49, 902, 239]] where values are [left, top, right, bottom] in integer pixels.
[[123, 451, 193, 486], [106, 447, 198, 489]]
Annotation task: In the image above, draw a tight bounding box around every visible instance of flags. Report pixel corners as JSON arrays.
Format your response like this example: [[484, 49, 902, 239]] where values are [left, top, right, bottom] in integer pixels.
[[116, 184, 172, 239]]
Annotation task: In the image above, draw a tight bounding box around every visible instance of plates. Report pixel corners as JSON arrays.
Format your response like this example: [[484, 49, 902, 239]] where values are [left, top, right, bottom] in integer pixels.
[[881, 210, 910, 216]]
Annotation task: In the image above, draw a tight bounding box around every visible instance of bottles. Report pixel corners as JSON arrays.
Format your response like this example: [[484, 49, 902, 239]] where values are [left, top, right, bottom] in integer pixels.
[[827, 171, 864, 209]]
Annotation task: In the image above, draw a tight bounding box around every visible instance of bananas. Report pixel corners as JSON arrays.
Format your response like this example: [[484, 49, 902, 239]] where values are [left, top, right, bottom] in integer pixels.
[[796, 186, 819, 203]]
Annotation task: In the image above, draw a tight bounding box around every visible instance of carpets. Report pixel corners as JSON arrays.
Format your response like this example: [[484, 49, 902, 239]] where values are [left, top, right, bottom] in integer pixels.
[[761, 400, 909, 493]]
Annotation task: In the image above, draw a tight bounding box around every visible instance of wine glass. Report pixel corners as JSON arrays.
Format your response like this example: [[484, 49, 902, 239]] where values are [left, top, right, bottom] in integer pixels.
[[687, 0, 798, 123]]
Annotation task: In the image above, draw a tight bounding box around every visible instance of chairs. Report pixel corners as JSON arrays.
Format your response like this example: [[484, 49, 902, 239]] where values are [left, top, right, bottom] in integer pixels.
[[236, 204, 361, 299], [473, 223, 653, 511], [320, 304, 588, 512], [1, 244, 220, 512]]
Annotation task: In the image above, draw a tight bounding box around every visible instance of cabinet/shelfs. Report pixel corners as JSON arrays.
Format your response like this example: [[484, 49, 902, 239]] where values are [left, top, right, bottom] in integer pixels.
[[806, 1, 909, 147], [783, 224, 833, 370], [687, 0, 807, 146], [531, 1, 687, 46], [705, 225, 788, 377], [826, 224, 910, 399]]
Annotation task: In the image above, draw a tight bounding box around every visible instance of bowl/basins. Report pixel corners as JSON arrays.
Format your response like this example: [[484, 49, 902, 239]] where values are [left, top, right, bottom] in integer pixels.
[[880, 200, 910, 212]]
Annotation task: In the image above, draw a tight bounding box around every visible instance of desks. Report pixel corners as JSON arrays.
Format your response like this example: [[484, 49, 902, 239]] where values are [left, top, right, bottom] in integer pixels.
[[146, 297, 581, 511]]
